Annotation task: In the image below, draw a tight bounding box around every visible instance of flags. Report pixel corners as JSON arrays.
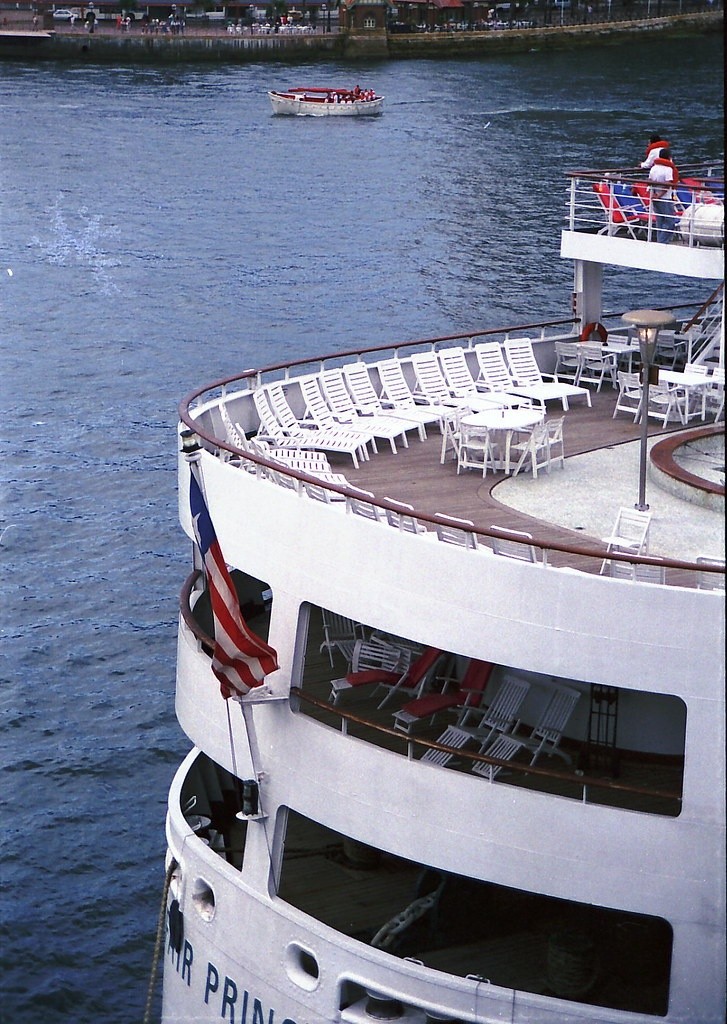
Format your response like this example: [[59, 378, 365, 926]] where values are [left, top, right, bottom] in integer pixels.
[[190, 464, 280, 699]]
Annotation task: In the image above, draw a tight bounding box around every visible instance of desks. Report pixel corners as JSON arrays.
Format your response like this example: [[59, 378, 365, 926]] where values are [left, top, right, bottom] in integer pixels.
[[425, 1010, 456, 1021], [186, 815, 211, 829], [656, 369, 713, 424], [462, 408, 546, 475], [661, 329, 709, 366], [568, 340, 640, 373], [365, 988, 393, 1001]]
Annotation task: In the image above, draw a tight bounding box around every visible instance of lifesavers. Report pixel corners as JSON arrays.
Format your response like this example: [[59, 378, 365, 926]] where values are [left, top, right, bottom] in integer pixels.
[[581, 320, 609, 342]]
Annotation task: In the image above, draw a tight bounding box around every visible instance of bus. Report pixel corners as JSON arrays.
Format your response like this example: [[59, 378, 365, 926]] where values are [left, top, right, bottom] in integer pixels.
[[62, 5, 149, 23]]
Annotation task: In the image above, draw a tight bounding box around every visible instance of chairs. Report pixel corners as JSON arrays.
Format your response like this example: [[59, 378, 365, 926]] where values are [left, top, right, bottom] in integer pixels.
[[217, 322, 726, 592], [465, 974, 491, 984], [321, 607, 581, 783], [591, 178, 726, 241], [404, 957, 424, 966]]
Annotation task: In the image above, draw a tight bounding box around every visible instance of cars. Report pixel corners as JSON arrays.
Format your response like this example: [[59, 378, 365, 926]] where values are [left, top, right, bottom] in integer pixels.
[[52, 9, 79, 21]]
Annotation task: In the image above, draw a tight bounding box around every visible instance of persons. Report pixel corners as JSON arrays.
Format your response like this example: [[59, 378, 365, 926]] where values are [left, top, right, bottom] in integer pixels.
[[227, 13, 301, 34], [415, 9, 537, 32], [326, 85, 376, 103], [115, 14, 185, 35], [649, 149, 675, 244], [639, 135, 672, 169]]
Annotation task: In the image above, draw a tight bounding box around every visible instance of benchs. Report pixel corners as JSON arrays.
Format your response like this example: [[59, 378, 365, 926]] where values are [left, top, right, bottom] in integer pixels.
[[213, 834, 226, 860]]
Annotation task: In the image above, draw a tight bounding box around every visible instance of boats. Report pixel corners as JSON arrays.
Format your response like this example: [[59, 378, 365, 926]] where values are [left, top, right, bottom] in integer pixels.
[[266, 86, 386, 117], [141, 157, 727, 1024]]
